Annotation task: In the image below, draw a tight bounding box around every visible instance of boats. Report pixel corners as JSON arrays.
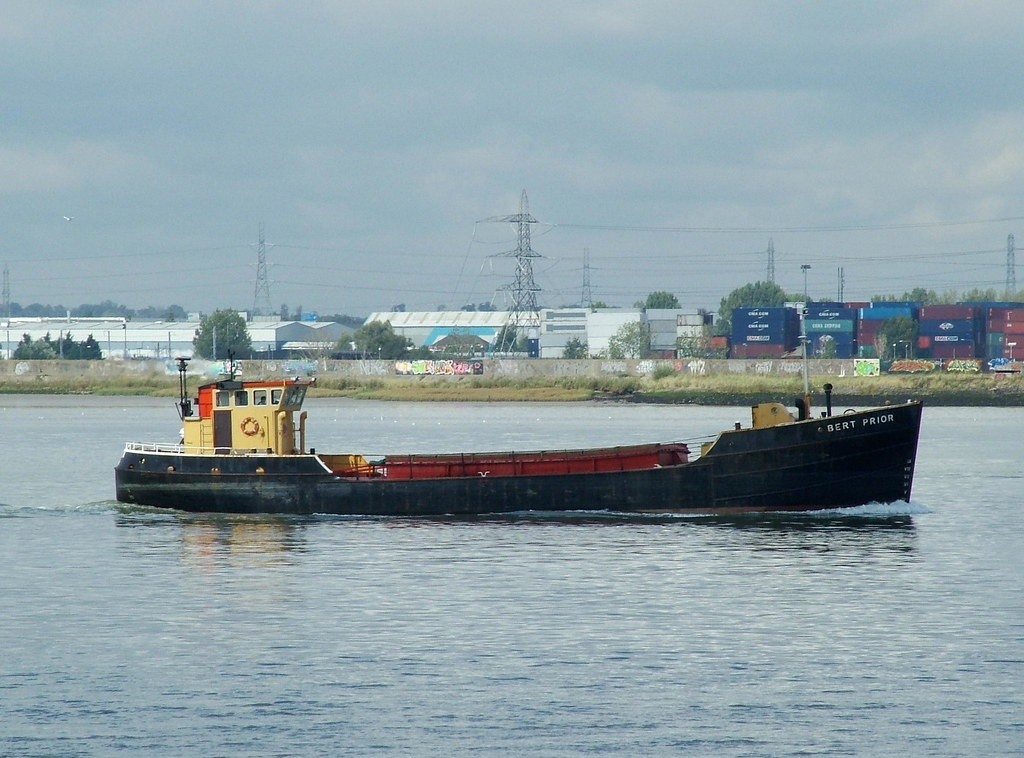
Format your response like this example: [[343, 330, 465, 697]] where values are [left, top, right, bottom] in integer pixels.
[[115, 341, 928, 521]]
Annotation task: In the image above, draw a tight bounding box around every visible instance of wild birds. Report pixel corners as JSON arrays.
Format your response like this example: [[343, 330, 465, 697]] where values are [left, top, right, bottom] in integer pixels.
[[63, 216, 77, 221]]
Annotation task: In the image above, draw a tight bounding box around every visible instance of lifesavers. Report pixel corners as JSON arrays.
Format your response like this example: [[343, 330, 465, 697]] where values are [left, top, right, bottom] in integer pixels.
[[240, 416, 260, 437]]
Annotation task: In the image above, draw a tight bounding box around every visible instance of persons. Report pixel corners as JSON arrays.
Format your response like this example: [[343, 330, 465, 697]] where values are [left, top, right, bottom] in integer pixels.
[[258, 396, 266, 405]]
